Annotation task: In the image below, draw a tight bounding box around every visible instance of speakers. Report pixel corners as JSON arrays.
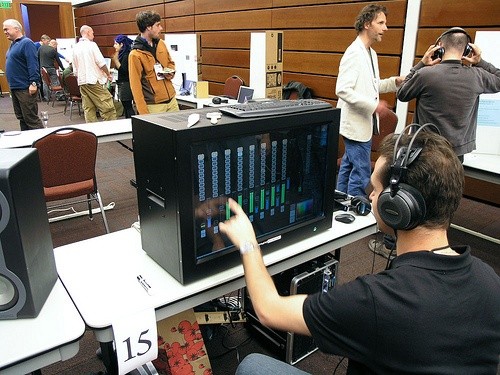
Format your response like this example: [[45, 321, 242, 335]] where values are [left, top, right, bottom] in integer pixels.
[[0, 147, 58, 320]]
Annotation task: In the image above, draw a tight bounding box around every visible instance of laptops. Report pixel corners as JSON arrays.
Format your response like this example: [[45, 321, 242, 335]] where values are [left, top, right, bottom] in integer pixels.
[[236, 86, 255, 104]]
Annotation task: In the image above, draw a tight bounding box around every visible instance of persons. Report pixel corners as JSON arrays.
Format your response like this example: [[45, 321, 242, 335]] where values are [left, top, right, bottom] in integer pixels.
[[72, 25, 117, 123], [335, 3, 406, 198], [3, 19, 45, 131], [396, 27, 500, 163], [33, 34, 70, 101], [218, 134, 500, 375], [63, 62, 76, 94], [38, 39, 65, 102], [112, 35, 133, 119], [128, 10, 181, 116]]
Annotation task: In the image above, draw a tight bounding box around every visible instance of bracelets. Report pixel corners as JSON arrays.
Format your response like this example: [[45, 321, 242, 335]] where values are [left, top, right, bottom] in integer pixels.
[[239, 241, 260, 254]]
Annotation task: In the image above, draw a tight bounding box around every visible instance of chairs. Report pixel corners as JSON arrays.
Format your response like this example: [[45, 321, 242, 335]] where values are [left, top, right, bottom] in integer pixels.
[[220, 75, 311, 100], [30, 67, 109, 234]]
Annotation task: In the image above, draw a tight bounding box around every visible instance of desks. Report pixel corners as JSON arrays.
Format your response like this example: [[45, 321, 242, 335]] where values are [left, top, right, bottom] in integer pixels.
[[0, 118, 137, 189], [0, 277, 86, 375], [173, 91, 237, 110], [53, 189, 380, 375]]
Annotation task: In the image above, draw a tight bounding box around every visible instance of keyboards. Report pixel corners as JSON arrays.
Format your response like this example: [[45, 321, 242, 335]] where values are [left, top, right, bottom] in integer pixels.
[[219, 98, 332, 118]]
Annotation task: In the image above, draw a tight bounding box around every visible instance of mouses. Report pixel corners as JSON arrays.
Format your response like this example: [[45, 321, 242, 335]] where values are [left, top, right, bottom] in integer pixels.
[[335, 214, 355, 223]]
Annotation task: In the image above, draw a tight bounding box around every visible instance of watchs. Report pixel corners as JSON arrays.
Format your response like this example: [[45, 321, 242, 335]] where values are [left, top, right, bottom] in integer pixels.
[[32, 81, 36, 86]]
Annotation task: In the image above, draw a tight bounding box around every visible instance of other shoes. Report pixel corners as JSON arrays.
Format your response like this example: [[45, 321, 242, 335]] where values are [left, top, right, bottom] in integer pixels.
[[368, 239, 397, 260]]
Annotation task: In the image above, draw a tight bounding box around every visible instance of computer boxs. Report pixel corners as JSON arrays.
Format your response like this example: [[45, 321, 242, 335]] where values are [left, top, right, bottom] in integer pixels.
[[241, 253, 338, 366]]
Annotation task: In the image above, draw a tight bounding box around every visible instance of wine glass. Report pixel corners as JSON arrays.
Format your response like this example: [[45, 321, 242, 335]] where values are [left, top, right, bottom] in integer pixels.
[[40, 110, 48, 131]]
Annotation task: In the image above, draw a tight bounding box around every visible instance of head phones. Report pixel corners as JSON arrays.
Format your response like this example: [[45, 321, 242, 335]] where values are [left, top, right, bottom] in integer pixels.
[[434, 29, 473, 58], [212, 97, 229, 104], [374, 123, 442, 231], [349, 196, 371, 216]]
